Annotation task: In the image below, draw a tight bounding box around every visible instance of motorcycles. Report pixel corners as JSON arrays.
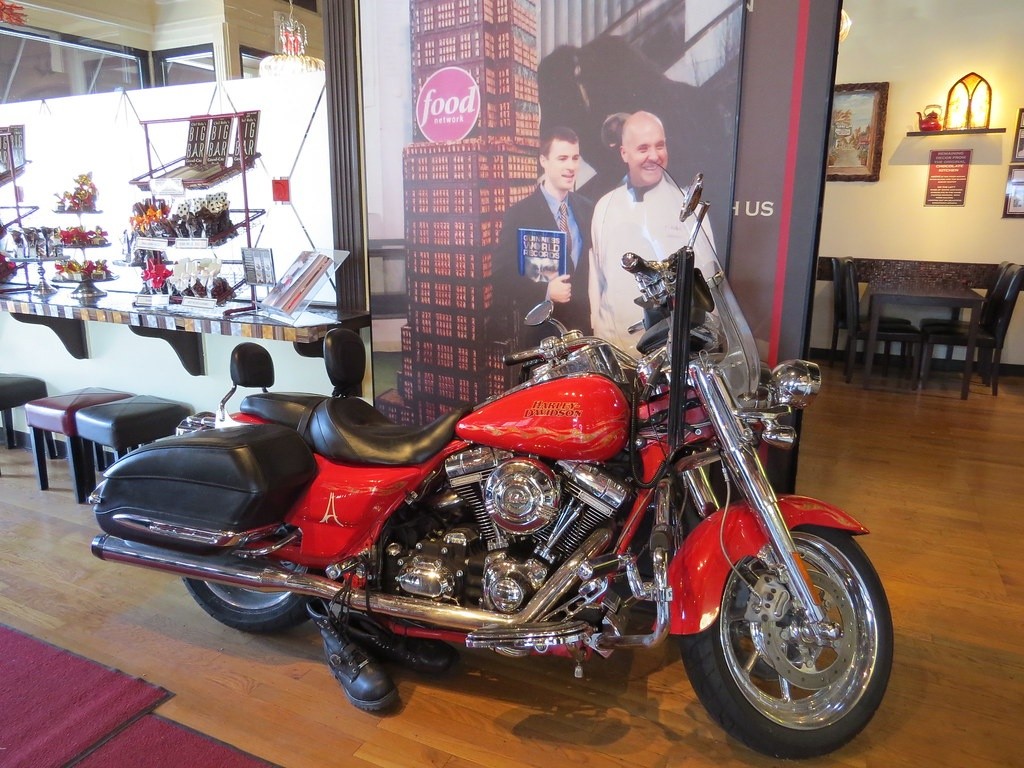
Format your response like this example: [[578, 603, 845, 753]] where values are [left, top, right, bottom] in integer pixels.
[[87, 159, 895, 762]]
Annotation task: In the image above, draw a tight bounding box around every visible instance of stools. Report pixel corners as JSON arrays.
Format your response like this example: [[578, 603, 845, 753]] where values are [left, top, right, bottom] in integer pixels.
[[0, 373, 56, 475], [23, 387, 196, 505]]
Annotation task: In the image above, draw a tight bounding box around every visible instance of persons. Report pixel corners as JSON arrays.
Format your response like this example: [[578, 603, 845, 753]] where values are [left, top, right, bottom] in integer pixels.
[[492, 110, 716, 357]]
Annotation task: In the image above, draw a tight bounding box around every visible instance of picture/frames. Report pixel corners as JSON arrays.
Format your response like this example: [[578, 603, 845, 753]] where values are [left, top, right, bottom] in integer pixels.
[[824, 81, 889, 182], [1002, 108, 1024, 218]]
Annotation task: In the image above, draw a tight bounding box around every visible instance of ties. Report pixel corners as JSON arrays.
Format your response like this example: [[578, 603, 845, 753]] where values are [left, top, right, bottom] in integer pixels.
[[558, 202, 572, 259]]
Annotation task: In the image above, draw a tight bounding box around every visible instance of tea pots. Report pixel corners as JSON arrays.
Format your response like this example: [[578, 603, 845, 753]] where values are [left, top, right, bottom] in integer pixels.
[[916, 105, 943, 131]]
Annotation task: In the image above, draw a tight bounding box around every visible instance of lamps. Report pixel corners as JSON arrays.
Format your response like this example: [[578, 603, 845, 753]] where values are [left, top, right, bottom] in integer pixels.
[[942, 72, 992, 131], [258, 0, 326, 77]]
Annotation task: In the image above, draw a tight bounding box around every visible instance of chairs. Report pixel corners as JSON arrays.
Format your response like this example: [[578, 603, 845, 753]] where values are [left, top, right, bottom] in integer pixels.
[[829, 257, 1024, 396]]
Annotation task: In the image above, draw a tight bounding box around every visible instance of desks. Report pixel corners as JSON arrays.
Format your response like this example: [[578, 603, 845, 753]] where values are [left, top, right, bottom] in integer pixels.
[[862, 278, 987, 401], [1, 281, 372, 377]]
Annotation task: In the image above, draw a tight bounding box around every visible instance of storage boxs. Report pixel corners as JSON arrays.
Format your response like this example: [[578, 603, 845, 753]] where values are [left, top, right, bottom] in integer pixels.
[[89, 423, 318, 549]]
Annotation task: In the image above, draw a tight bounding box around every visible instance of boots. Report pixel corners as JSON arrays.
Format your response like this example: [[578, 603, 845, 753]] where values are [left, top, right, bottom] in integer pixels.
[[305, 597, 399, 711], [338, 611, 460, 677]]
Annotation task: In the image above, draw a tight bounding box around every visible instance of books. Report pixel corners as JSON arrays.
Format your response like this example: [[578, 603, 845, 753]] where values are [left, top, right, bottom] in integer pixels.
[[517, 228, 567, 284], [262, 251, 331, 315]]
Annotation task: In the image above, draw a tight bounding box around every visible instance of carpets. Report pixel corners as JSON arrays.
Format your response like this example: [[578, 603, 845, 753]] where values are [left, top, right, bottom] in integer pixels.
[[69, 712, 286, 767], [0, 622, 176, 768]]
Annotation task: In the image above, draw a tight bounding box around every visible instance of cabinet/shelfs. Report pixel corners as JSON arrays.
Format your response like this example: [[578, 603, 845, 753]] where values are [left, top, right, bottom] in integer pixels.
[[129, 113, 266, 316], [1, 131, 41, 294]]
